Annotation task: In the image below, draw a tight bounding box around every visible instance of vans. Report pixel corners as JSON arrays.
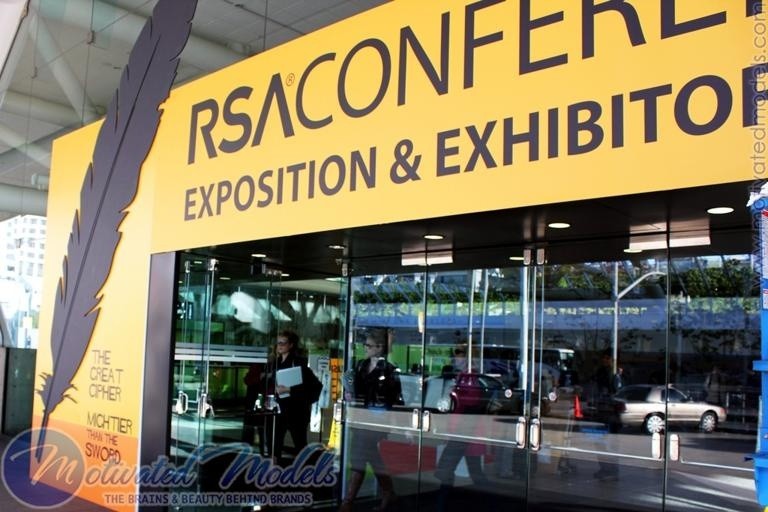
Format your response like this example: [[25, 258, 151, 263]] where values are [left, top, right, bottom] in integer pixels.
[[579, 366, 708, 424]]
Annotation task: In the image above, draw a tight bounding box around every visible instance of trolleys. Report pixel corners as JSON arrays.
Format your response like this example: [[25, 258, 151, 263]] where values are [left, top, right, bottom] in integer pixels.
[[557, 403, 577, 476]]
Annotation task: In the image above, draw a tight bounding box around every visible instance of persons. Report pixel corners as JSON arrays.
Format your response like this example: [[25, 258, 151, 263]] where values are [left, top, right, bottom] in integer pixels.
[[203, 328, 626, 512]]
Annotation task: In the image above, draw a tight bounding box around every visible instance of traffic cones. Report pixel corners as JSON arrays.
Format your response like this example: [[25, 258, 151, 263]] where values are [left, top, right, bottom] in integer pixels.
[[572, 395, 583, 418]]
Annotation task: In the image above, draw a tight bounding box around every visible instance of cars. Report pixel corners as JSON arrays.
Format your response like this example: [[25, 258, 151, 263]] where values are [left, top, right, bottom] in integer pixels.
[[173, 374, 203, 394], [343, 356, 571, 419], [594, 383, 727, 433]]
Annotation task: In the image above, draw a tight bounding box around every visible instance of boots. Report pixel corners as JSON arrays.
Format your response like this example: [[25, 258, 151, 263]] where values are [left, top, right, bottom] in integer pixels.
[[373, 475, 399, 511], [337, 471, 362, 512]]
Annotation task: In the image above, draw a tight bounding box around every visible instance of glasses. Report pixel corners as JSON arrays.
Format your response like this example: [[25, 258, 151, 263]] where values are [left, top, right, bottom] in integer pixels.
[[277, 340, 287, 345]]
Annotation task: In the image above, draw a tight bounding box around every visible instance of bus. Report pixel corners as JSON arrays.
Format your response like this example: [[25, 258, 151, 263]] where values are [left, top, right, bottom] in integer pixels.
[[420, 343, 579, 389]]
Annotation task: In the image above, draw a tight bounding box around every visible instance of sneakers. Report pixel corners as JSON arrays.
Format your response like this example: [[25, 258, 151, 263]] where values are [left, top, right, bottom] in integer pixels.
[[594, 471, 617, 483]]
